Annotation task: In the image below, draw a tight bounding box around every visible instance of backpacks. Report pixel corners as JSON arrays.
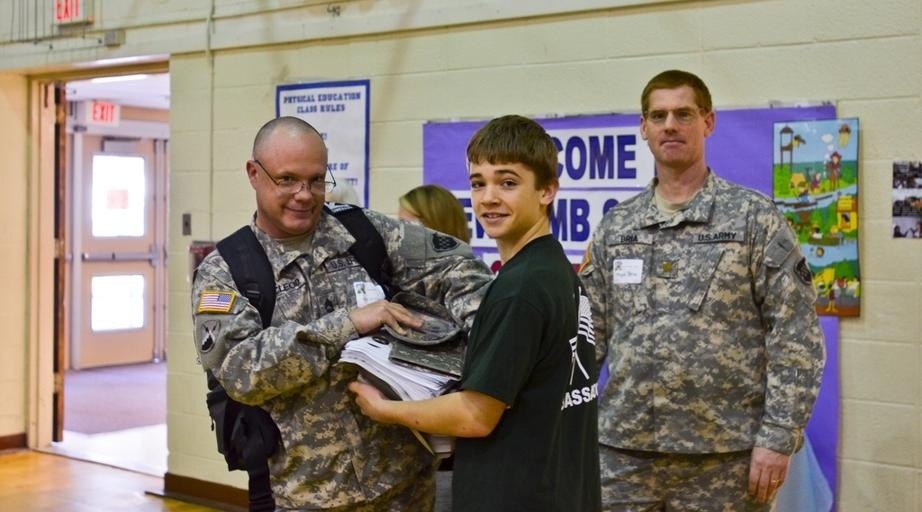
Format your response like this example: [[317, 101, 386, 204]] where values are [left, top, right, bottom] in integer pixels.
[[206, 367, 284, 471]]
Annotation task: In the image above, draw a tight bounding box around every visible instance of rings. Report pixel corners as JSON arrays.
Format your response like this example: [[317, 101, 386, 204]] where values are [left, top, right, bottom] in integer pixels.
[[770, 481, 780, 487]]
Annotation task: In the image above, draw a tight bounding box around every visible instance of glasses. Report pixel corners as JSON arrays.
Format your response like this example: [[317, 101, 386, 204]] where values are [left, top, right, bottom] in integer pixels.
[[254, 159, 336, 197]]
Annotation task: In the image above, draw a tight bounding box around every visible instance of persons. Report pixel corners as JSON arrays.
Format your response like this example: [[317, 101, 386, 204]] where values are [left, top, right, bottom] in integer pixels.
[[189, 116, 499, 511], [576, 68, 826, 511], [346, 115, 603, 511], [399, 183, 473, 247], [894, 171, 921, 237]]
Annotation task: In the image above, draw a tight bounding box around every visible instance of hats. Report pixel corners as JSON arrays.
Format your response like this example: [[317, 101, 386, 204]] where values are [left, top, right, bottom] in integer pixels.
[[383, 289, 468, 349]]
